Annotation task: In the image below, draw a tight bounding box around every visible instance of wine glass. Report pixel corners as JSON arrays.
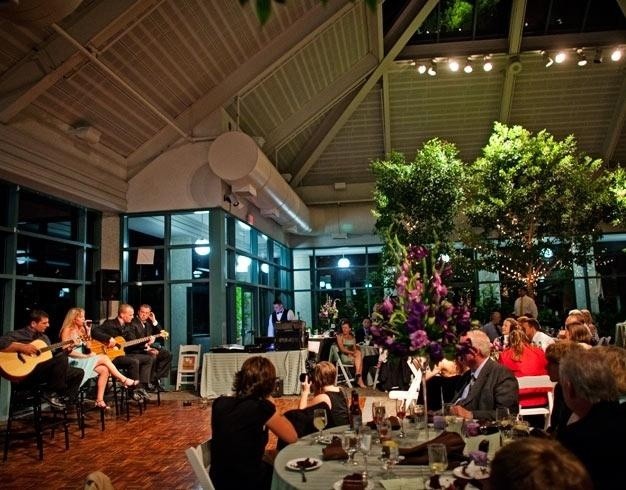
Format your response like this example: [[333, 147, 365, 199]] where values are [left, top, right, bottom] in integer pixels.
[[370, 401, 386, 423], [376, 422, 392, 443], [412, 404, 424, 431], [512, 420, 530, 441], [494, 407, 510, 427], [380, 442, 397, 478], [355, 426, 372, 478], [341, 426, 360, 467], [427, 443, 450, 475], [310, 408, 327, 441], [394, 398, 406, 437], [442, 404, 457, 430]]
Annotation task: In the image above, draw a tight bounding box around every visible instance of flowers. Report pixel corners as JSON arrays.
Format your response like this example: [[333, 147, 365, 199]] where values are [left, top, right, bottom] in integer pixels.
[[361, 225, 479, 372], [317, 294, 341, 321]]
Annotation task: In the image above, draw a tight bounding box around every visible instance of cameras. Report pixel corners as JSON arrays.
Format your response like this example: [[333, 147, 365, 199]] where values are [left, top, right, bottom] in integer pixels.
[[300, 369, 315, 382]]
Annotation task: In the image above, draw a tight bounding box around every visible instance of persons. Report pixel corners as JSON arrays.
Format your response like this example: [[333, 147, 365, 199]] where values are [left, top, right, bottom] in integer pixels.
[[335, 285, 626, 432], [57, 306, 141, 411], [549, 342, 626, 488], [488, 433, 593, 490], [297, 358, 353, 430], [0, 307, 85, 414], [266, 298, 296, 350], [131, 303, 173, 394], [207, 355, 301, 490], [90, 301, 155, 402]]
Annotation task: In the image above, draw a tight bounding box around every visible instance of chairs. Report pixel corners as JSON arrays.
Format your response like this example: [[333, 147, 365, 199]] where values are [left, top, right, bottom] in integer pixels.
[[329, 344, 355, 389], [3, 368, 161, 461], [185, 439, 216, 490], [516, 375, 554, 432]]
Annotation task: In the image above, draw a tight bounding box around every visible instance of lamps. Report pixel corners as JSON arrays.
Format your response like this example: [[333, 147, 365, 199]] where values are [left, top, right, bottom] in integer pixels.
[[236, 228, 253, 267], [408, 44, 623, 77], [337, 253, 349, 269], [194, 214, 210, 256], [260, 239, 269, 274]]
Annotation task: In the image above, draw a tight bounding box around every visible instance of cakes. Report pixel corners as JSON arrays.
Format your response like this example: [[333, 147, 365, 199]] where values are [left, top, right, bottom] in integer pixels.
[[344, 473, 363, 490], [463, 460, 476, 476], [429, 474, 453, 489]]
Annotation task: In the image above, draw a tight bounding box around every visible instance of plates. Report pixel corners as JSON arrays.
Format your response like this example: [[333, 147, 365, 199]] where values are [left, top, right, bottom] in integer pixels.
[[331, 477, 375, 489], [283, 456, 322, 472], [424, 478, 466, 489], [451, 465, 492, 479], [319, 434, 343, 445]]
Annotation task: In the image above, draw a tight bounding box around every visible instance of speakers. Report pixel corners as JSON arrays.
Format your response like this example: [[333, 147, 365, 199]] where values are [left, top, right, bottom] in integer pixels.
[[95, 268, 122, 302]]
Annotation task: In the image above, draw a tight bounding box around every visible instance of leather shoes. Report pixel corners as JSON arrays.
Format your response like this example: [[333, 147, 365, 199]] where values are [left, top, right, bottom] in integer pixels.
[[43, 393, 63, 407], [131, 379, 170, 400]]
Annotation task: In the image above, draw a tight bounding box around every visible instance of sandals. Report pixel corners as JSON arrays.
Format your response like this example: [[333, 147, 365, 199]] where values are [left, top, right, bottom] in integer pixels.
[[95, 400, 110, 410], [123, 378, 139, 389]]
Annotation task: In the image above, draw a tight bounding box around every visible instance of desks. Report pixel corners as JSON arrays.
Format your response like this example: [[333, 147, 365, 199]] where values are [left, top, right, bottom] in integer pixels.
[[200, 349, 309, 400]]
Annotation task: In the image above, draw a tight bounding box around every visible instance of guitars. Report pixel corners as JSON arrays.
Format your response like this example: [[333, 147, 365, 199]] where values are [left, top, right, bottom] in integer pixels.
[[0, 340, 74, 378], [84, 330, 170, 361]]
